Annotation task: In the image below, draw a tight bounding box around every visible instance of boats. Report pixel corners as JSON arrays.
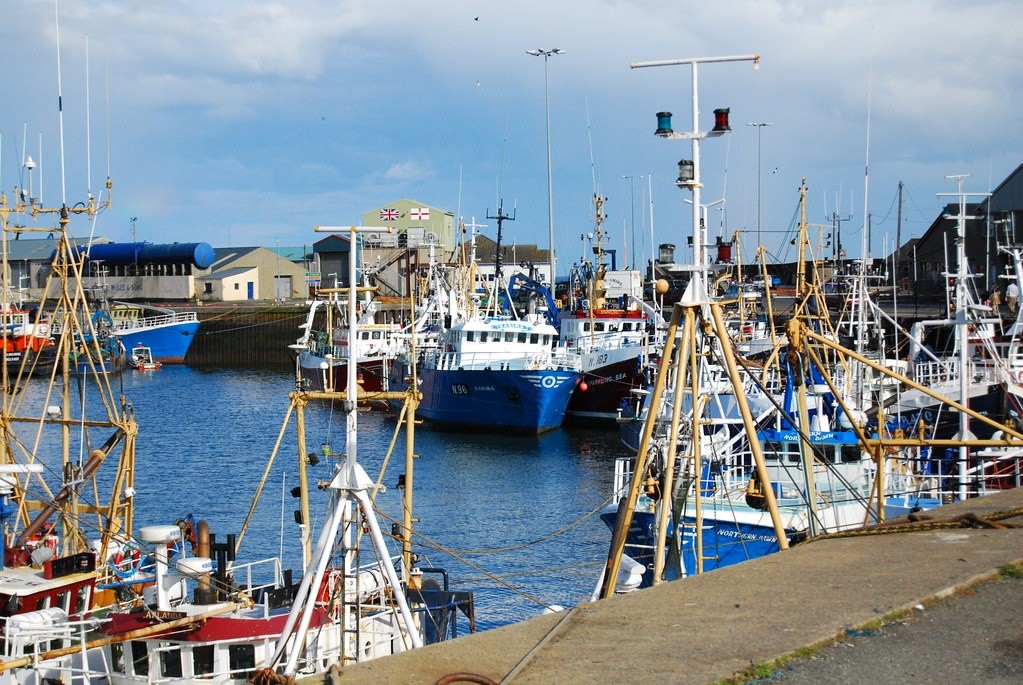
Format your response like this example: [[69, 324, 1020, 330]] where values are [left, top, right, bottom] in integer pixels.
[[599, 56, 1023, 588], [0, 260, 206, 377], [0, 0, 479, 685], [289, 99, 653, 439]]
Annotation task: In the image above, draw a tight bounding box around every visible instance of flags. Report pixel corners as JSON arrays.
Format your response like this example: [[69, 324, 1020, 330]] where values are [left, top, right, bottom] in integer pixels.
[[411, 207, 430, 220], [380, 208, 399, 221]]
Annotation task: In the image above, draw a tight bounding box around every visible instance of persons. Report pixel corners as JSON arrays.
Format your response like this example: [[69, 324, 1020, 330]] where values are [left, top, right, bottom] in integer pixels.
[[1005, 280, 1018, 318], [990, 281, 1002, 314]]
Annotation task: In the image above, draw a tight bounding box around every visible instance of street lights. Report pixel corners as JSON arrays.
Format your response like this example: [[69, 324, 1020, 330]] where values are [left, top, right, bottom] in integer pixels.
[[526, 46, 568, 302]]
